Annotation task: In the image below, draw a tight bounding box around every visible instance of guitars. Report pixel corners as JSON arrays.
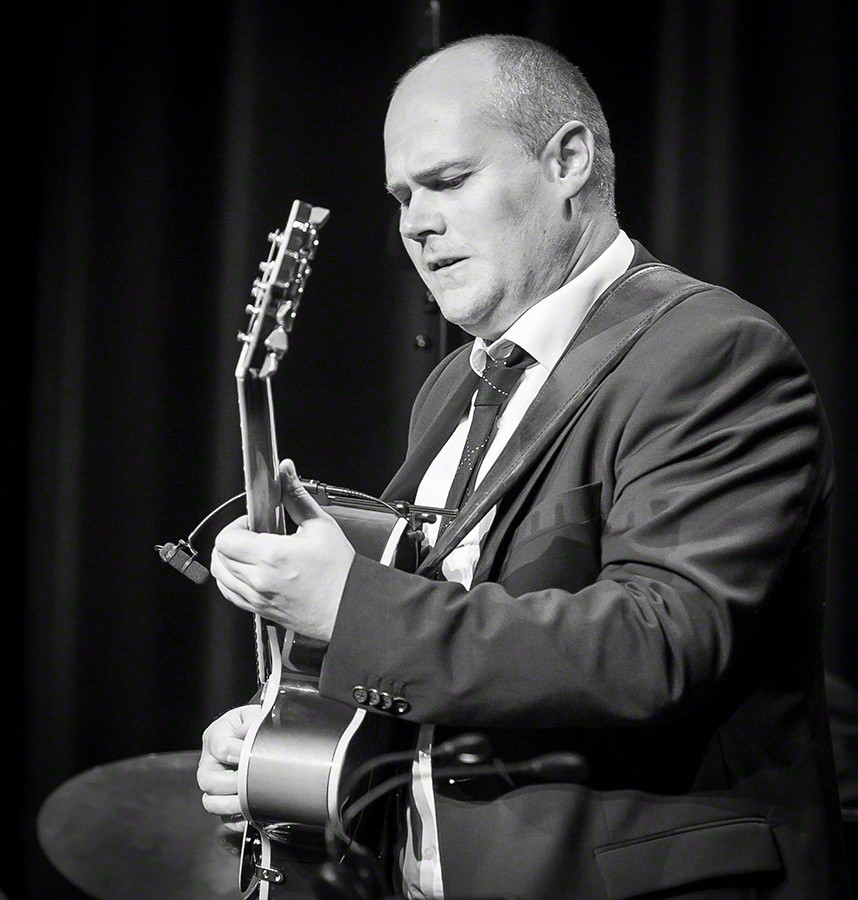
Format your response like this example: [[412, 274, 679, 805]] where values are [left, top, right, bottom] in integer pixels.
[[236, 198, 408, 900]]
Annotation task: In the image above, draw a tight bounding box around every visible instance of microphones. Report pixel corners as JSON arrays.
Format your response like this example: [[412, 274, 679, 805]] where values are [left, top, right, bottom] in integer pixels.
[[160, 542, 208, 584]]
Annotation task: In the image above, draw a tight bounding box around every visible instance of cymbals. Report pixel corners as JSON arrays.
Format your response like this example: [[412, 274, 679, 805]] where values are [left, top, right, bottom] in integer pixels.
[[39, 750, 237, 900]]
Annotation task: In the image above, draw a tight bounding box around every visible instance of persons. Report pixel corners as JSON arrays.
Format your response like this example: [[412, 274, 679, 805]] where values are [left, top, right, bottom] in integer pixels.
[[196, 34, 858, 900]]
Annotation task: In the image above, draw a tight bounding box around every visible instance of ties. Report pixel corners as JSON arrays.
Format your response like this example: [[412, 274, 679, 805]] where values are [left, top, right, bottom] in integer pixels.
[[392, 343, 539, 837]]
